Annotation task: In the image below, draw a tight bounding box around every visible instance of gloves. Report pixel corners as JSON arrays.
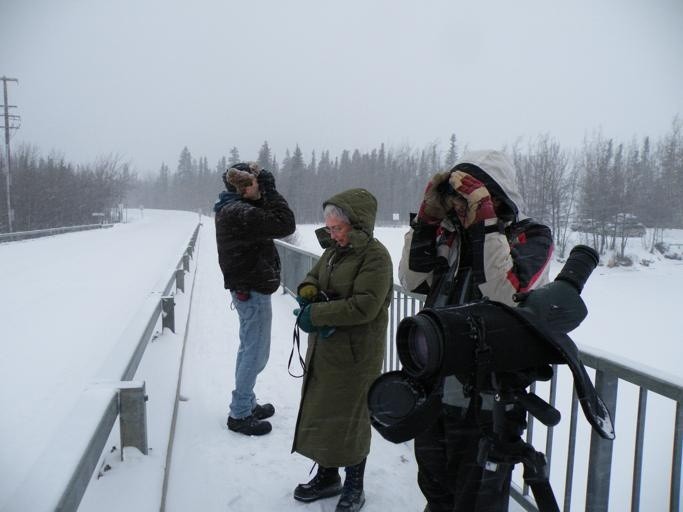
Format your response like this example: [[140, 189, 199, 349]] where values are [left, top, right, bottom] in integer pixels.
[[417, 181, 444, 226], [299, 285, 318, 302], [293, 305, 318, 333], [449, 169, 496, 230]]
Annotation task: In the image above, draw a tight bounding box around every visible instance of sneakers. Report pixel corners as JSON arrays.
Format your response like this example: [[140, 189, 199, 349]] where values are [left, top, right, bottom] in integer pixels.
[[228, 414, 272, 435], [252, 404, 274, 420]]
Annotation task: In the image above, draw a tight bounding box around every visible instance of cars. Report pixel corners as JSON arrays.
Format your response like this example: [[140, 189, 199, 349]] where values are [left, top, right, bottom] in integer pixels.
[[569, 211, 648, 238]]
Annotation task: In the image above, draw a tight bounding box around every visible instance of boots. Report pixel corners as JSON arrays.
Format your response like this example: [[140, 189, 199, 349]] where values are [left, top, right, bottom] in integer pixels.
[[336, 459, 366, 511], [294, 465, 341, 502]]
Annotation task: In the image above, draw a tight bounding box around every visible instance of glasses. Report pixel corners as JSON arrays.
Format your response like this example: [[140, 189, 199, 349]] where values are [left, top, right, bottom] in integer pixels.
[[323, 225, 345, 234]]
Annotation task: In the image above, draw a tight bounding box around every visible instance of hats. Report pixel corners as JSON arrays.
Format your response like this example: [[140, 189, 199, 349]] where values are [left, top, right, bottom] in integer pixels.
[[226, 168, 254, 196]]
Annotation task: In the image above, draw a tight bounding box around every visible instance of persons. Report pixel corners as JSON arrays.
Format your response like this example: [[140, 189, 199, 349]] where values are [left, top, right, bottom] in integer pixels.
[[293, 187, 394, 512], [399, 147, 556, 512], [214, 163, 296, 436]]
